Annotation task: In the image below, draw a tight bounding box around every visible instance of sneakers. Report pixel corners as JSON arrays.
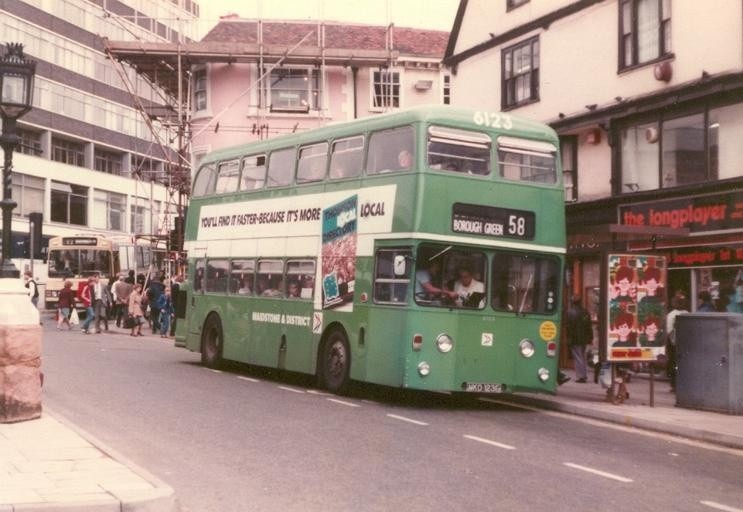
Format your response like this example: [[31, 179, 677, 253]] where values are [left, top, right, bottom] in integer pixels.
[[129, 332, 145, 337], [556, 373, 587, 385]]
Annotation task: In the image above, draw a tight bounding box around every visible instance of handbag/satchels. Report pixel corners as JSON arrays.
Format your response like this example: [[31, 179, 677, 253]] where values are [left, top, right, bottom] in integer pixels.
[[123, 314, 140, 328]]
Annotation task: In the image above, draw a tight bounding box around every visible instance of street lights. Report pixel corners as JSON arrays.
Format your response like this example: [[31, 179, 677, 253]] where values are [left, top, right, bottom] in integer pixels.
[[0, 41, 38, 277]]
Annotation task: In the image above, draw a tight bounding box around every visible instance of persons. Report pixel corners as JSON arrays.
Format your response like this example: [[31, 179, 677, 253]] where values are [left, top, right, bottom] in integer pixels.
[[396, 150, 412, 169], [22, 269, 43, 327], [54, 250, 182, 339], [696, 290, 719, 313], [192, 263, 315, 300], [565, 293, 594, 383], [414, 259, 459, 304], [555, 368, 570, 386], [664, 291, 693, 394], [451, 267, 488, 309]]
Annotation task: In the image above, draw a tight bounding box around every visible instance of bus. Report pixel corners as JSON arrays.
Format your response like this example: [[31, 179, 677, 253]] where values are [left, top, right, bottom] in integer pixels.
[[44, 234, 172, 320], [174, 102, 568, 397]]
